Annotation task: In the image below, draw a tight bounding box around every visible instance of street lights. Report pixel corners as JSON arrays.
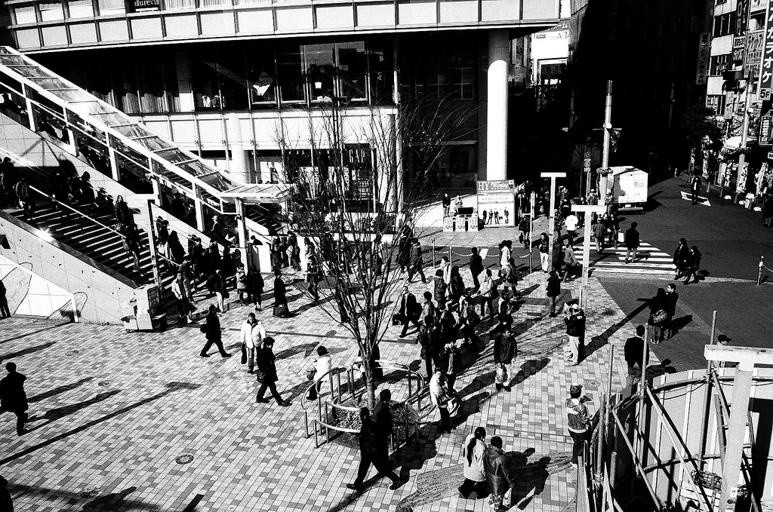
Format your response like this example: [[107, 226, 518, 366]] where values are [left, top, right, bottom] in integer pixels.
[[539, 171, 567, 278], [569, 203, 609, 317], [327, 95, 353, 274], [718, 67, 753, 195]]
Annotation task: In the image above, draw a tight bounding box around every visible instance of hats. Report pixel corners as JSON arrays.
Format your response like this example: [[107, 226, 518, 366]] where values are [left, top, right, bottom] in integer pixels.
[[718, 335, 731, 342], [209, 305, 218, 312]]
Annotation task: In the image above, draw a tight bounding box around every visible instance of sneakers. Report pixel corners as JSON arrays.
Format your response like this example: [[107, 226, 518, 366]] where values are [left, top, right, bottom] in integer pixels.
[[278, 401, 292, 406], [389, 480, 402, 490], [200, 353, 210, 357], [496, 383, 502, 391], [399, 333, 407, 338], [347, 483, 364, 491], [17, 428, 27, 436], [257, 399, 269, 403], [222, 353, 232, 357], [306, 396, 317, 401], [505, 386, 511, 392], [458, 486, 470, 499]]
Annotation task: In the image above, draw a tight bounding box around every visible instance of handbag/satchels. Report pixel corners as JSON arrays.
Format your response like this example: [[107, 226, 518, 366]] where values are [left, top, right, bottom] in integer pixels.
[[241, 344, 248, 364], [392, 313, 407, 326], [467, 307, 480, 327], [491, 285, 500, 300], [306, 367, 317, 381], [507, 273, 517, 284], [651, 309, 669, 324], [446, 398, 460, 418], [200, 324, 208, 333], [257, 371, 268, 385]]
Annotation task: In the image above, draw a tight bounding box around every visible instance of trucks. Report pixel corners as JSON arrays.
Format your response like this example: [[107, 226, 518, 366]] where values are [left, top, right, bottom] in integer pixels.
[[595, 163, 650, 213]]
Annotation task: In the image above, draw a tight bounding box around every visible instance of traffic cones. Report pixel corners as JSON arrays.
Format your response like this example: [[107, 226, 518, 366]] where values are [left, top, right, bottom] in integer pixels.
[[673, 166, 738, 204]]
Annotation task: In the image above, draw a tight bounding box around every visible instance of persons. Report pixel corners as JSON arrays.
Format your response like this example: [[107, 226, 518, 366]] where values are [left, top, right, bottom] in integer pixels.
[[546, 271, 561, 317], [306, 345, 332, 401], [256, 336, 292, 406], [457, 427, 514, 511], [483, 207, 509, 224], [1, 362, 29, 436], [347, 389, 403, 490], [1, 477, 16, 511], [2, 93, 384, 319], [745, 192, 771, 227], [673, 238, 701, 284], [624, 325, 649, 377], [690, 177, 703, 206], [442, 193, 462, 216], [240, 313, 267, 373], [648, 283, 679, 345], [565, 385, 593, 466], [200, 305, 232, 358], [397, 226, 523, 434], [710, 335, 732, 369], [564, 303, 584, 366], [516, 182, 641, 281]]
